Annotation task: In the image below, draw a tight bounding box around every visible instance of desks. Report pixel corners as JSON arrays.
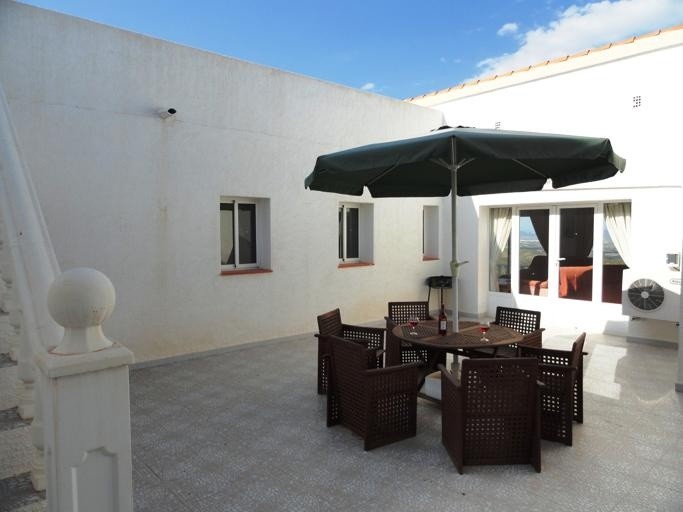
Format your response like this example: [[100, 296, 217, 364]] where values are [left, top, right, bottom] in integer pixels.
[[393, 317, 525, 399]]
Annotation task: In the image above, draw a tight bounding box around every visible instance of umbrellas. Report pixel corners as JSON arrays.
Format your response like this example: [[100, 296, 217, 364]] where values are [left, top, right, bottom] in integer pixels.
[[305, 123, 626, 333]]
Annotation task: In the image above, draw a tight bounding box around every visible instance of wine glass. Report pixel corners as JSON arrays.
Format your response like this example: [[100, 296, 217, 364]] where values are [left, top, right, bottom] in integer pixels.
[[479, 320, 490, 342], [407, 315, 419, 336]]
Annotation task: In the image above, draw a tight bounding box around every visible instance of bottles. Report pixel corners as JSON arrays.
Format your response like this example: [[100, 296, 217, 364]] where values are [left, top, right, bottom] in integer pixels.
[[438, 303, 447, 335]]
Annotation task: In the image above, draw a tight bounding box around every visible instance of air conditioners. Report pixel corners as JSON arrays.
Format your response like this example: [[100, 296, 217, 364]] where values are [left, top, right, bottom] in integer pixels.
[[620, 269, 681, 324]]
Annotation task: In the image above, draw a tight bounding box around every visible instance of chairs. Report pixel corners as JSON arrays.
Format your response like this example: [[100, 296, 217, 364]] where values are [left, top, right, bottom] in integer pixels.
[[384, 300, 437, 368], [519, 331, 588, 448], [437, 356, 547, 475], [314, 307, 388, 398], [314, 332, 428, 453], [469, 305, 546, 358]]
